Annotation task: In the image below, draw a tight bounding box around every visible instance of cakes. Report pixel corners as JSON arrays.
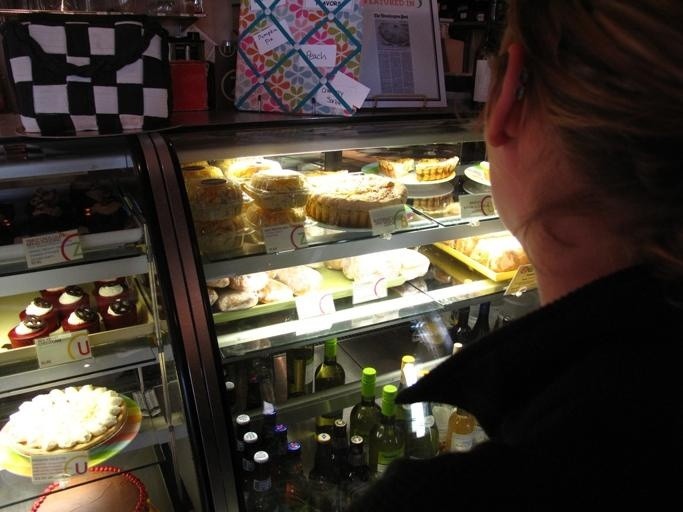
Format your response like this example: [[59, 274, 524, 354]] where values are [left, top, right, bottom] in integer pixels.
[[8, 276, 137, 349], [33, 466, 149, 512], [24, 184, 124, 233], [305, 170, 408, 228], [8, 385, 129, 463], [379, 156, 459, 212]]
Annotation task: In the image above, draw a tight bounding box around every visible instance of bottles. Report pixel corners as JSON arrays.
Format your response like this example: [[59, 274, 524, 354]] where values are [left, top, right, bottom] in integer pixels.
[[246, 358, 276, 406], [275, 441, 310, 512], [431, 400, 487, 456], [261, 404, 280, 452], [240, 431, 259, 497], [350, 355, 438, 476], [247, 451, 275, 512], [452, 302, 491, 342], [269, 423, 288, 473], [235, 413, 250, 463], [307, 419, 370, 512], [312, 338, 346, 429], [285, 345, 307, 399]]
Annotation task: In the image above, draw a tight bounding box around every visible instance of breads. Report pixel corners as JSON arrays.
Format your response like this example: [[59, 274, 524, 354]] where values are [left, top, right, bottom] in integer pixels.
[[323, 248, 430, 286], [443, 229, 530, 272], [206, 261, 324, 312], [183, 156, 310, 256], [479, 161, 491, 181]]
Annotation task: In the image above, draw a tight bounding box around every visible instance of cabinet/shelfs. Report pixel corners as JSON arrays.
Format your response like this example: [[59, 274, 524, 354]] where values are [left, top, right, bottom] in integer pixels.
[[0, 114, 542, 512]]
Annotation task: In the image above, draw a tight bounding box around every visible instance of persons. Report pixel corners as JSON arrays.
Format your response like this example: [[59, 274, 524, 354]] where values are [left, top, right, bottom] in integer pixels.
[[354, 0, 681, 508]]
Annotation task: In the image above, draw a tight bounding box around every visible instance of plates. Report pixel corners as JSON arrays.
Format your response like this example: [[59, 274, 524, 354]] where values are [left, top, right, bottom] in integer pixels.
[[464, 165, 490, 186], [0, 387, 144, 478], [361, 161, 456, 185], [463, 180, 475, 194], [406, 182, 454, 199], [315, 222, 371, 232]]
